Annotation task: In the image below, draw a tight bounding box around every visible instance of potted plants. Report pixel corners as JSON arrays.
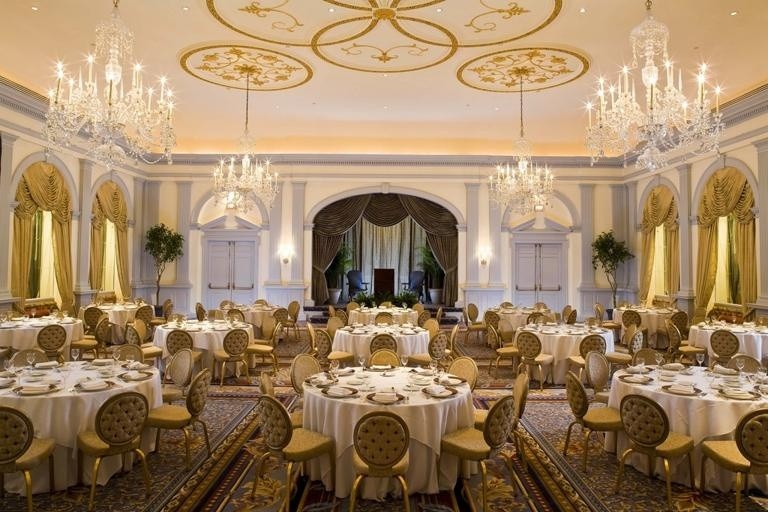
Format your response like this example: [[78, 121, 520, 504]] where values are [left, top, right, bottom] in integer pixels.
[[143, 222, 184, 317], [593, 230, 634, 321], [413, 243, 443, 305], [327, 241, 357, 303]]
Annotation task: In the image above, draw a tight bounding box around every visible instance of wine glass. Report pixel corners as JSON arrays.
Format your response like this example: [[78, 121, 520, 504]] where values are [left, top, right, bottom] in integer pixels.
[[705, 372, 714, 392], [71, 349, 79, 368], [113, 352, 120, 368], [735, 359, 745, 379], [26, 353, 35, 372], [3, 360, 14, 378], [636, 358, 644, 374], [330, 360, 339, 373], [703, 314, 763, 330], [401, 355, 408, 371], [125, 355, 134, 371], [0, 307, 75, 328], [695, 354, 704, 371], [654, 353, 662, 371], [493, 303, 542, 312], [163, 314, 247, 329], [756, 368, 766, 386], [429, 360, 437, 377], [357, 355, 364, 370], [360, 301, 407, 311]]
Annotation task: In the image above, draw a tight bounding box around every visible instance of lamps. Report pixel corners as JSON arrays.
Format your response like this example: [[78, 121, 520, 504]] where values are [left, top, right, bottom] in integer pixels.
[[210, 67, 282, 211], [488, 69, 554, 214], [587, 0, 725, 172], [480, 248, 488, 265], [43, 1, 177, 170], [280, 245, 290, 263]]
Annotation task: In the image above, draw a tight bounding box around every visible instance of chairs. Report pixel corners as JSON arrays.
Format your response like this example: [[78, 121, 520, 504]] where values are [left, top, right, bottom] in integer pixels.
[[402, 271, 427, 303], [346, 269, 368, 302]]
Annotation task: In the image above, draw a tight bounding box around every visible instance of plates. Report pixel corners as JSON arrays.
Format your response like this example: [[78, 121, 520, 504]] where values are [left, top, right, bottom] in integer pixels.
[[342, 323, 424, 335], [121, 362, 154, 371], [324, 369, 354, 376], [365, 365, 394, 371], [520, 323, 607, 336], [0, 379, 16, 389], [366, 393, 404, 404], [75, 380, 114, 392], [421, 387, 458, 396], [618, 375, 653, 384], [321, 387, 357, 396], [662, 385, 701, 396], [624, 366, 652, 374], [433, 377, 466, 386], [12, 386, 60, 396], [117, 372, 153, 381], [33, 362, 63, 370], [717, 389, 761, 401], [304, 377, 338, 388]]
